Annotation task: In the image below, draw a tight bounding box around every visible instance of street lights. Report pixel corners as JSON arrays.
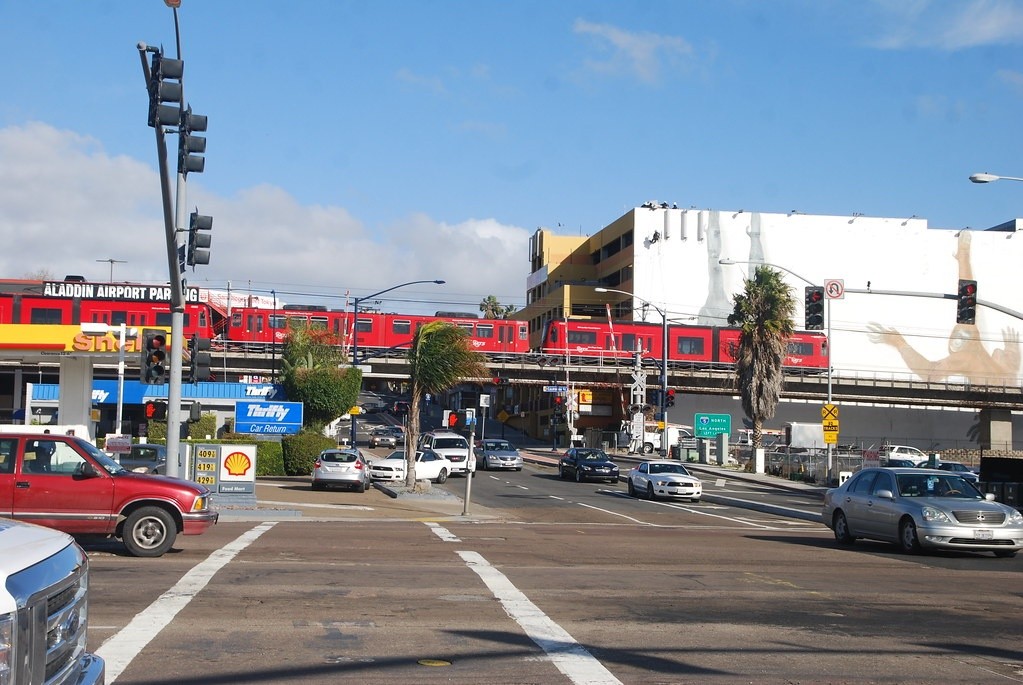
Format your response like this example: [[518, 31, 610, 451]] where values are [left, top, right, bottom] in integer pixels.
[[350, 280, 445, 449], [720, 258, 831, 481], [595, 287, 668, 457], [81, 322, 125, 462]]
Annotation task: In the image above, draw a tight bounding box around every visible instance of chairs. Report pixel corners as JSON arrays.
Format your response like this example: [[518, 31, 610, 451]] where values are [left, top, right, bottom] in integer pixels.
[[326, 453, 336, 460], [347, 455, 352, 460]]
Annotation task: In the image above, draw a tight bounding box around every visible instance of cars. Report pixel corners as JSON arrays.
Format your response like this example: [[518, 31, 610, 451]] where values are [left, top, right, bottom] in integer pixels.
[[111, 443, 166, 475], [821, 467, 1022, 558], [0, 431, 219, 557], [558, 443, 980, 501], [0, 516, 106, 685], [311, 401, 524, 493]]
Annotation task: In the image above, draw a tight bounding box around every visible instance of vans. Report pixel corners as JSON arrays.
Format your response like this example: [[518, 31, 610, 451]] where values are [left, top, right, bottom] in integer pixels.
[[622, 421, 785, 454]]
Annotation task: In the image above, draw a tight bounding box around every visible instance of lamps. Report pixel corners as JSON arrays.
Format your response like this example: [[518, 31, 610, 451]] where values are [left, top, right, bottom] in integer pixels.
[[962, 226, 971, 231], [650, 230, 660, 243], [738, 208, 743, 214], [687, 205, 696, 212], [791, 209, 796, 214], [1014, 228, 1023, 232], [910, 214, 917, 219]]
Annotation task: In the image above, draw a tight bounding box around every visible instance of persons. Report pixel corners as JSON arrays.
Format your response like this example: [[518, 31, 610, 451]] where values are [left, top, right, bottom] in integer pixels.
[[37, 430, 56, 470]]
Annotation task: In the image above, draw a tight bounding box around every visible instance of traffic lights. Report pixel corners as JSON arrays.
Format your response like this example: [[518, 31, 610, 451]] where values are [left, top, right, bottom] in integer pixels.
[[448, 376, 566, 429], [628, 403, 651, 414], [141, 328, 167, 386], [805, 287, 824, 330], [652, 389, 676, 407], [144, 402, 166, 420], [957, 280, 978, 325], [188, 336, 212, 382], [154, 44, 214, 267]]
